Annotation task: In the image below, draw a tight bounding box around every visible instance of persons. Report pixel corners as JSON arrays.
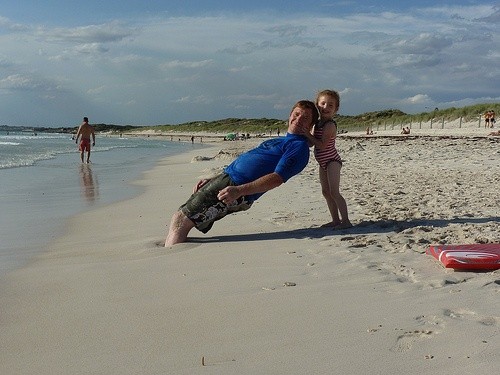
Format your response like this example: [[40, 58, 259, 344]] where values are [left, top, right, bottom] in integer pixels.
[[484, 110, 495, 128], [336, 126, 411, 135], [165, 99, 319, 248], [104, 126, 282, 144], [299, 89, 353, 230], [75, 116, 95, 164]]
